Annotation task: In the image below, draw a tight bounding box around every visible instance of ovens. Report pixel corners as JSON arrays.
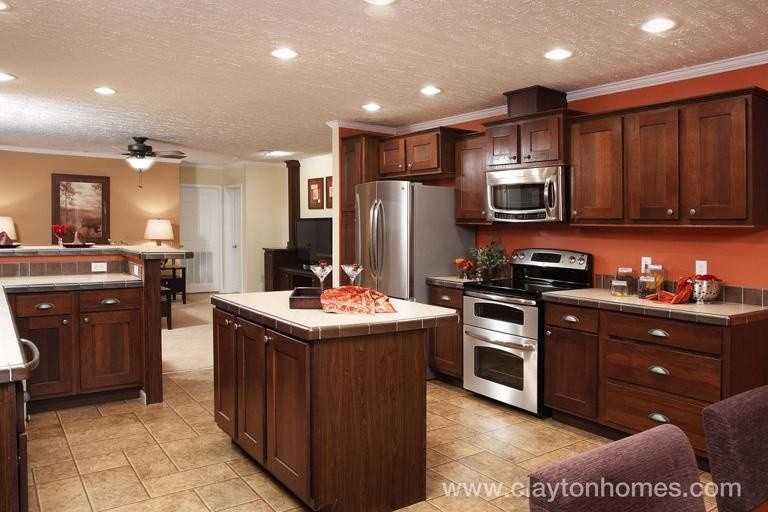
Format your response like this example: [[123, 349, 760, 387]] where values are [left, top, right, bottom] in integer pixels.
[[462, 295, 538, 415]]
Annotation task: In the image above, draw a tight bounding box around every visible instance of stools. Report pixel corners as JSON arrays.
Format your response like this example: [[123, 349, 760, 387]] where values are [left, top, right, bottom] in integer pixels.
[[160, 285, 172, 330]]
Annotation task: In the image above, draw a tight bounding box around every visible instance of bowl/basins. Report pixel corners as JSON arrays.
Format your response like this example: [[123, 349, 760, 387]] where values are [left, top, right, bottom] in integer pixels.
[[687, 279, 721, 305]]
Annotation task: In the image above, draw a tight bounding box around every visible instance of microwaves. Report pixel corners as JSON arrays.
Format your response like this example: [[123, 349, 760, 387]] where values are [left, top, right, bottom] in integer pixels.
[[485, 164, 565, 223]]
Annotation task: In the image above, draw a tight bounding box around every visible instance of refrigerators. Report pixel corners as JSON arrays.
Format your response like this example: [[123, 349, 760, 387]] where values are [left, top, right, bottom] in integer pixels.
[[355, 181, 476, 380]]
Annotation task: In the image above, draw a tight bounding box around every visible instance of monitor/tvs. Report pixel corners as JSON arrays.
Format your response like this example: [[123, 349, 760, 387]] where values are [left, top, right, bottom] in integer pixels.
[[293, 218, 331, 268]]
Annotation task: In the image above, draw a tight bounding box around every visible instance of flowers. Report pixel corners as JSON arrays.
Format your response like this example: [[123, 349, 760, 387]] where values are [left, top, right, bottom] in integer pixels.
[[453, 259, 473, 273], [50, 223, 68, 238]]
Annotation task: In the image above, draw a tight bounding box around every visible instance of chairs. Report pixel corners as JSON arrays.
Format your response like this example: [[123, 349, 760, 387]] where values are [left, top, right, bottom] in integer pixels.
[[702, 384, 767, 512], [528, 422, 705, 511]]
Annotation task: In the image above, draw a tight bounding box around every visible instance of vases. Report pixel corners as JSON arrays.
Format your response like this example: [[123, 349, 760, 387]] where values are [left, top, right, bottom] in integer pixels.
[[55, 238, 65, 248], [459, 272, 470, 279]]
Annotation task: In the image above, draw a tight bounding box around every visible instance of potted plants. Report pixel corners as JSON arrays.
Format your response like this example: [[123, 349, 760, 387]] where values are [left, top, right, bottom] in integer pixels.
[[468, 240, 511, 281]]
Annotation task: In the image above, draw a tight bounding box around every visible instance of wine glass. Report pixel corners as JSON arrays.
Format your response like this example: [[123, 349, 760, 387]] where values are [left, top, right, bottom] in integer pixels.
[[311, 263, 334, 291], [340, 263, 364, 286], [52, 224, 69, 249]]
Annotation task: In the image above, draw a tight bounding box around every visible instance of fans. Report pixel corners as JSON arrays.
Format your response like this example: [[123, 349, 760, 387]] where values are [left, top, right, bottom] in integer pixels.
[[84, 136, 187, 160]]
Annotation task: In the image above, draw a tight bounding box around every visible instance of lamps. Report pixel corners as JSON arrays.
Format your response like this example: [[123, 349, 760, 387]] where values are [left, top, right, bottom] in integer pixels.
[[143, 219, 174, 246], [0, 216, 20, 249], [125, 157, 157, 188]]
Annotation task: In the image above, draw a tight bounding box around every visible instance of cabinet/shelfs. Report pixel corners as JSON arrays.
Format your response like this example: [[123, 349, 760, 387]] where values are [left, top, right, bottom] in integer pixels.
[[425, 275, 463, 388], [262, 245, 332, 292], [453, 85, 767, 230], [209, 288, 458, 512], [376, 125, 440, 180], [0, 245, 193, 512], [538, 287, 768, 459]]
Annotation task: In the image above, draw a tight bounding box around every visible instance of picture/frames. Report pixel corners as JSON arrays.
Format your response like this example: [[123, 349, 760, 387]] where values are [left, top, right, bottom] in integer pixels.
[[325, 176, 332, 209], [50, 173, 110, 245], [306, 177, 323, 209]]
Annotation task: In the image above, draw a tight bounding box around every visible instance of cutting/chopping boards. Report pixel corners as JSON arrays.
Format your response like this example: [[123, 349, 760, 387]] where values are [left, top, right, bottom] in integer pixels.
[[464, 248, 592, 296]]
